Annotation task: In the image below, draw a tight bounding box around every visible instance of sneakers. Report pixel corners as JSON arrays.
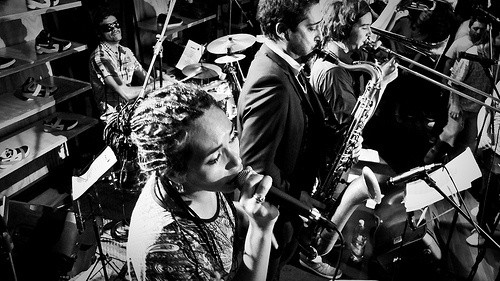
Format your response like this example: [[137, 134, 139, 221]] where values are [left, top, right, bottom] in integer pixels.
[[34, 31, 70, 55], [0, 57, 16, 69], [26, 0, 59, 10], [0, 145, 29, 169], [43, 115, 78, 133], [300, 258, 342, 279], [20, 77, 57, 100]]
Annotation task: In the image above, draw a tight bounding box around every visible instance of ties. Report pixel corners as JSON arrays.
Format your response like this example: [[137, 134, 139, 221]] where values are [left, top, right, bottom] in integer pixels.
[[297, 73, 307, 91]]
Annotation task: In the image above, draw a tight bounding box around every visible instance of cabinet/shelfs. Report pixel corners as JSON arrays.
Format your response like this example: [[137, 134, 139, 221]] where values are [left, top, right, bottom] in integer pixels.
[[0, 0, 98, 179], [135, 0, 220, 85]]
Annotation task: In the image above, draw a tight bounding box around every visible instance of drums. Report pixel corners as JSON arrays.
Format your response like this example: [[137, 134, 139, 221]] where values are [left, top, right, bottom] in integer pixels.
[[199, 81, 238, 120]]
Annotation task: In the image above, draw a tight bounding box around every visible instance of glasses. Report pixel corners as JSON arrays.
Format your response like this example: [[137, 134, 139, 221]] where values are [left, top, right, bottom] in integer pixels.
[[101, 21, 120, 32]]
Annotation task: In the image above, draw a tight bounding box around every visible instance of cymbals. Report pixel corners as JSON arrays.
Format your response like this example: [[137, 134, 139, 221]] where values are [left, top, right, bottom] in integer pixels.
[[207, 34, 256, 54], [214, 54, 246, 64], [182, 63, 222, 78]]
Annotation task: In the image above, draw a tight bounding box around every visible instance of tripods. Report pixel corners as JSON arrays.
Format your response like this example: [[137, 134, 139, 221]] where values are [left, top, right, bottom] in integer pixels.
[[71, 144, 123, 281]]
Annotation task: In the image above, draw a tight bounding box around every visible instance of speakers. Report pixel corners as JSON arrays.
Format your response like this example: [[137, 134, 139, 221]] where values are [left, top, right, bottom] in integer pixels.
[[7, 199, 104, 278], [370, 188, 443, 278]]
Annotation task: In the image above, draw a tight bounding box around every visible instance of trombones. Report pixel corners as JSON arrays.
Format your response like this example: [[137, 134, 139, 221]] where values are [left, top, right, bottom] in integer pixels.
[[363, 23, 500, 115]]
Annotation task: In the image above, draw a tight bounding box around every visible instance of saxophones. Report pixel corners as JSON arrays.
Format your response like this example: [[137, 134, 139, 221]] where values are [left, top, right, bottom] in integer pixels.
[[304, 46, 382, 256]]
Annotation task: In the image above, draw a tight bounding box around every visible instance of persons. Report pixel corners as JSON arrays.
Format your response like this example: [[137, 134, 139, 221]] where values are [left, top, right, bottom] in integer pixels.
[[102, 84, 280, 281], [236, 0, 500, 278], [89, 14, 154, 127]]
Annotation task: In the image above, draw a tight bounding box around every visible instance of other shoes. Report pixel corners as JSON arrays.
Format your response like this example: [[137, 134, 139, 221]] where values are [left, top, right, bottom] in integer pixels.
[[466, 230, 485, 246], [424, 146, 438, 163], [157, 14, 183, 30]]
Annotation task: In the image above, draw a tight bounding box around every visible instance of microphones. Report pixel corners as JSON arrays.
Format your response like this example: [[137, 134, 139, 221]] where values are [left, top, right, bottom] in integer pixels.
[[236, 170, 338, 231], [459, 51, 500, 65], [470, 2, 500, 23], [388, 163, 444, 192]]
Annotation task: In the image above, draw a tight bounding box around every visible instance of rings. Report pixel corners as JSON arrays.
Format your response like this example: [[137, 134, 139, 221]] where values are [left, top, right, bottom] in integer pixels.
[[253, 195, 264, 203]]
